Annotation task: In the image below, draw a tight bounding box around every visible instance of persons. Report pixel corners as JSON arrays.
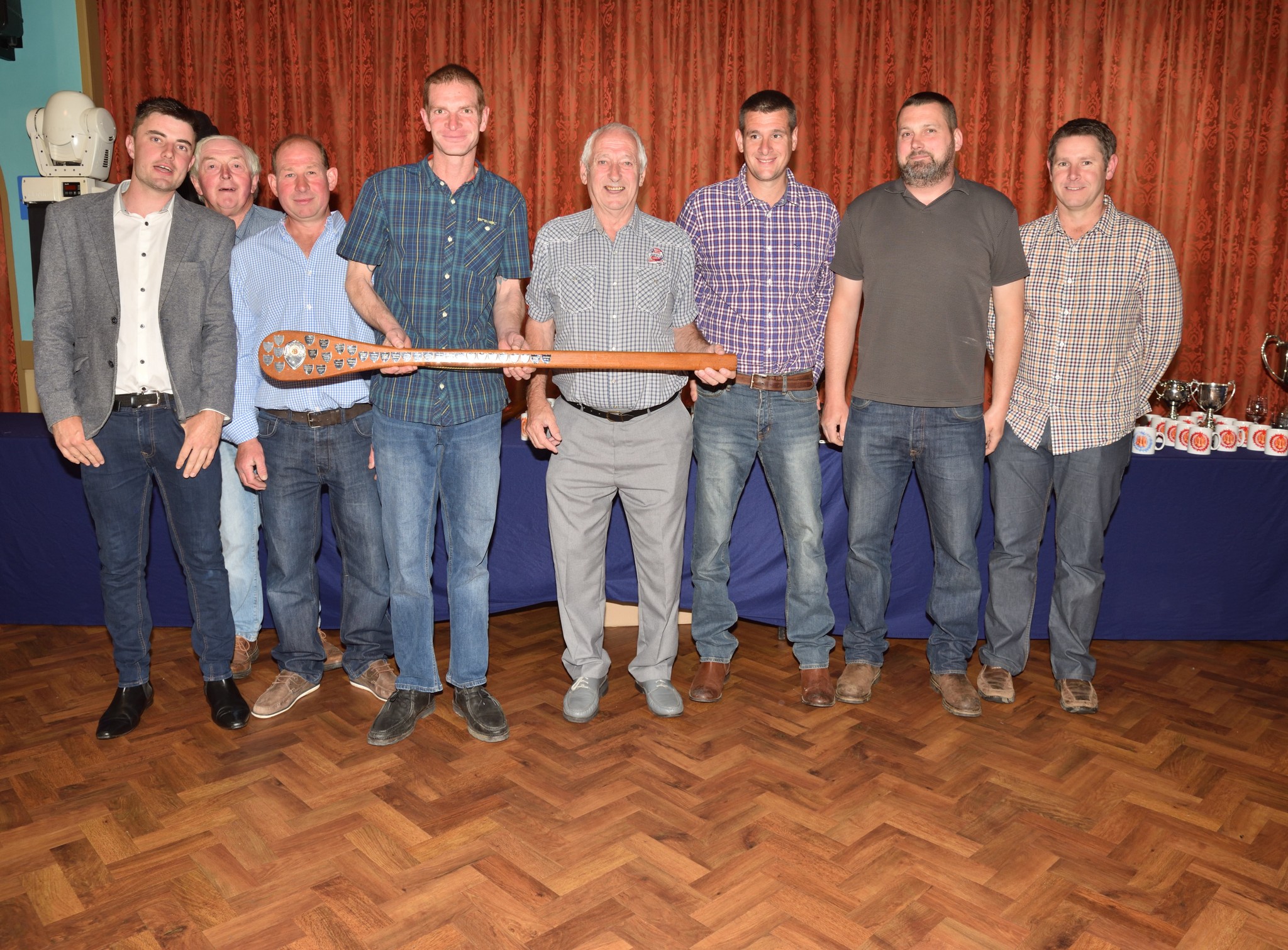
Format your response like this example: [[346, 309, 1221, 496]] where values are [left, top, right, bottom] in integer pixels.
[[222, 133, 398, 718], [820, 92, 1030, 716], [676, 90, 841, 706], [34, 96, 250, 738], [186, 136, 344, 679], [525, 122, 736, 723], [336, 63, 536, 746], [976, 118, 1182, 714]]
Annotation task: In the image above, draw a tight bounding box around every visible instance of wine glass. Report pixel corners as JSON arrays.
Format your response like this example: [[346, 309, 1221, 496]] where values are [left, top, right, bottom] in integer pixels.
[[1245, 395, 1268, 424]]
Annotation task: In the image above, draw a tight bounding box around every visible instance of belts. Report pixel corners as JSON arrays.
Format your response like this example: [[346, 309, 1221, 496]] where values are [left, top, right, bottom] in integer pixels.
[[560, 391, 683, 424], [261, 403, 374, 429], [733, 371, 820, 391], [108, 391, 175, 413]]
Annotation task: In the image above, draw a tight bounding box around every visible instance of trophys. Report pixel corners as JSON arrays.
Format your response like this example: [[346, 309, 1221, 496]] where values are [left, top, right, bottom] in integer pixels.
[[1155, 380, 1235, 433], [1261, 332, 1288, 429]]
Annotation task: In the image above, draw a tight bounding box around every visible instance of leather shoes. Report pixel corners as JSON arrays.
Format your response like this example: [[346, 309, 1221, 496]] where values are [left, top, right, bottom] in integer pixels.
[[800, 668, 835, 707], [929, 671, 981, 717], [203, 677, 251, 731], [635, 679, 684, 717], [452, 684, 509, 742], [96, 679, 154, 740], [690, 661, 731, 703], [367, 689, 436, 746], [835, 661, 883, 705], [563, 675, 610, 723]]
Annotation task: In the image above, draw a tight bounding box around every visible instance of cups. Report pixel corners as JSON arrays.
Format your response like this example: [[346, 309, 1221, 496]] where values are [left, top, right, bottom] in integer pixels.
[[1131, 411, 1288, 455]]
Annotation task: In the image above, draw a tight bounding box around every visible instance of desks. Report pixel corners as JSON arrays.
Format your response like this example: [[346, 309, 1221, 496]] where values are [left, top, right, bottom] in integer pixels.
[[0, 414, 1288, 643]]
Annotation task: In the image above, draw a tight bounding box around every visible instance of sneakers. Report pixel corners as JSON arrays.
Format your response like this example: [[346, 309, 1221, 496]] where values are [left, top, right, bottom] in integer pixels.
[[250, 670, 320, 720], [350, 659, 398, 702], [977, 663, 1016, 704], [315, 627, 345, 672], [1055, 678, 1098, 714], [228, 635, 261, 678]]
